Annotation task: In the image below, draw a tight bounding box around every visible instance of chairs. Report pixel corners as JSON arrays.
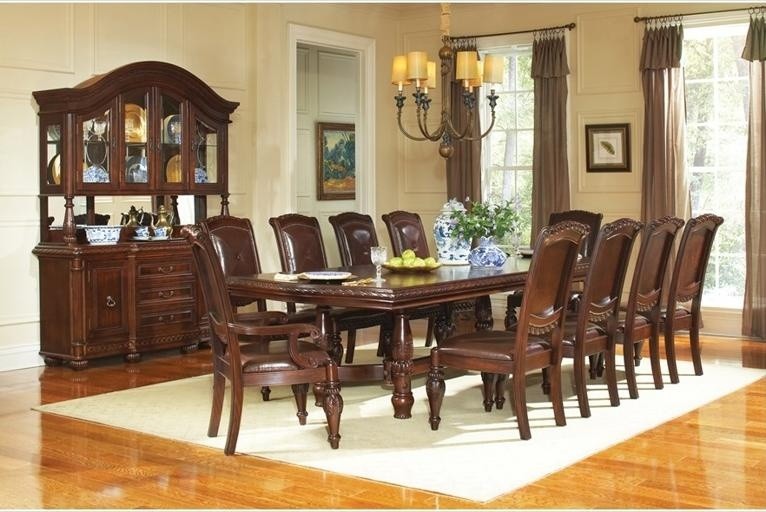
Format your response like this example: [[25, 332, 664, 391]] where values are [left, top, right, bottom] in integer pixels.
[[509, 218, 641, 419], [268, 214, 388, 366], [179, 227, 344, 456], [427, 220, 587, 438], [502, 209, 604, 338], [600, 215, 685, 392], [617, 213, 725, 376], [328, 211, 415, 356], [381, 211, 447, 346], [202, 214, 345, 401]]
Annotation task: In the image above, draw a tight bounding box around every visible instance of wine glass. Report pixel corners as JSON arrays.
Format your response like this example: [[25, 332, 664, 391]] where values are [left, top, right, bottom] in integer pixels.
[[370, 244, 388, 284], [94, 120, 106, 140]]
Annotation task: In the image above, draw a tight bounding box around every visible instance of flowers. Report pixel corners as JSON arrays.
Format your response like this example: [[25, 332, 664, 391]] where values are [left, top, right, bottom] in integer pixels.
[[445, 190, 526, 243]]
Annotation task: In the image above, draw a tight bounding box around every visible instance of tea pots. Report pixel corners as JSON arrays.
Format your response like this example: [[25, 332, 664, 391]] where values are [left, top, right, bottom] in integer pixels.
[[121, 204, 144, 227], [148, 206, 175, 237]]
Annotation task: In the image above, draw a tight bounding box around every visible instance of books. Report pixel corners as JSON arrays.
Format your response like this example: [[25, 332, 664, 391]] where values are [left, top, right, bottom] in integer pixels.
[[275, 270, 353, 283]]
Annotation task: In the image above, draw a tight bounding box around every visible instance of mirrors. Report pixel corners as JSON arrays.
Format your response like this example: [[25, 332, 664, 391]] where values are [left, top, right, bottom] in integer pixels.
[[46, 194, 197, 229]]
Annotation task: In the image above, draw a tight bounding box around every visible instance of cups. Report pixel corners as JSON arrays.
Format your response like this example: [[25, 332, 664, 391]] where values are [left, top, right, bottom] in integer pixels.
[[195, 168, 205, 184], [151, 226, 168, 237], [134, 225, 150, 238]]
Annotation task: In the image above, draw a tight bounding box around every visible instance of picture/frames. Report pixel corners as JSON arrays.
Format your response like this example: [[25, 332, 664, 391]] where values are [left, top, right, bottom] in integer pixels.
[[583, 122, 634, 174], [315, 121, 356, 201]]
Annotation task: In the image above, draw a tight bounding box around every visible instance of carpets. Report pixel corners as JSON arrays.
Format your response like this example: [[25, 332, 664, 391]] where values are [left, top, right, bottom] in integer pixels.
[[29, 340, 764, 509]]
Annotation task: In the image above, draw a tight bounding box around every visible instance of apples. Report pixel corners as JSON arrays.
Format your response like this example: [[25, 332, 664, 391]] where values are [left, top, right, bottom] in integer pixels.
[[390, 249, 435, 267]]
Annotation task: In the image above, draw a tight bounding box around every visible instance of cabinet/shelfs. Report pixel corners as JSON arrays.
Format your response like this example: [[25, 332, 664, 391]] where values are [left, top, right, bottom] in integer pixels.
[[30, 61, 240, 246], [130, 249, 193, 355], [33, 251, 131, 372]]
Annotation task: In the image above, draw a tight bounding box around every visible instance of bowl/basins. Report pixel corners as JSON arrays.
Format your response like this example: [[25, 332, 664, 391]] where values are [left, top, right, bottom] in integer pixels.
[[84, 225, 124, 246]]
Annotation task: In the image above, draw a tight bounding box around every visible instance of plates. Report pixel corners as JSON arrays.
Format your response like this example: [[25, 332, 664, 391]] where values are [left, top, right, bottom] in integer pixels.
[[165, 155, 182, 182], [48, 151, 88, 184], [302, 270, 352, 280], [132, 236, 149, 241], [163, 114, 181, 143], [381, 263, 442, 272], [82, 163, 108, 183], [150, 236, 169, 241], [48, 126, 60, 142], [125, 103, 147, 142], [125, 154, 147, 183], [517, 248, 535, 258]]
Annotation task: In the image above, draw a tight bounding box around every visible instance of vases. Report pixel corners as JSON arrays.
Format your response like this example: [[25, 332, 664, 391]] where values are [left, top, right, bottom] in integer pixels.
[[467, 234, 506, 268]]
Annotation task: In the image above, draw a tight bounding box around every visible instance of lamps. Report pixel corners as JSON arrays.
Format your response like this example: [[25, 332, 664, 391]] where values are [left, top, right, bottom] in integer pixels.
[[389, 4, 506, 158]]
